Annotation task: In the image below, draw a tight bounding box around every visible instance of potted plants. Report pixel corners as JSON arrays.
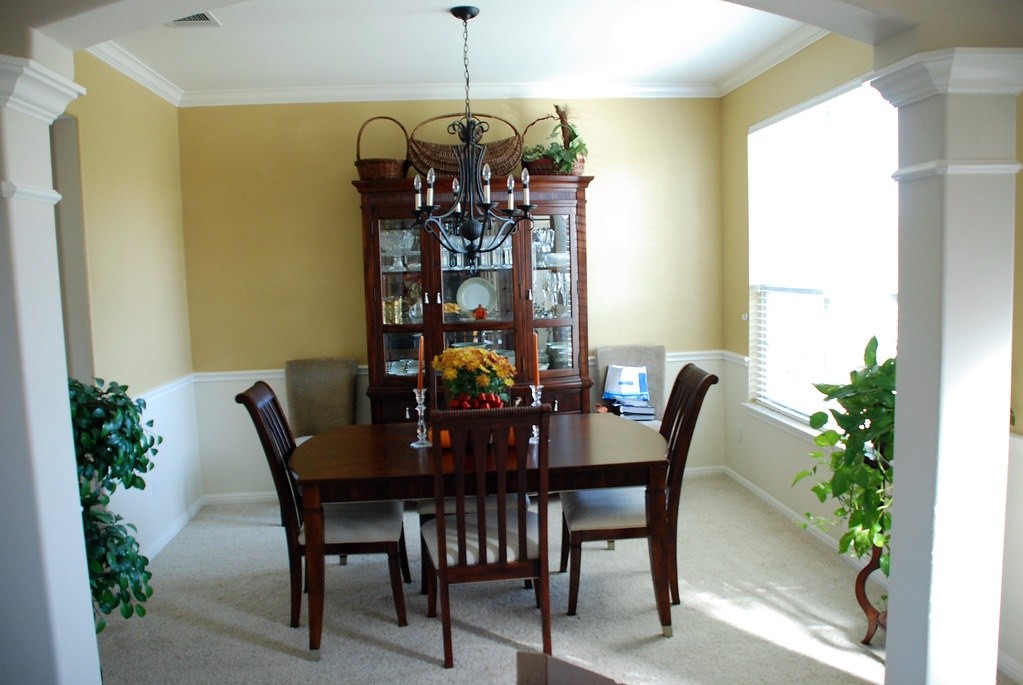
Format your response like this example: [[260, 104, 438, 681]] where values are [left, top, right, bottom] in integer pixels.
[[791, 335, 901, 576]]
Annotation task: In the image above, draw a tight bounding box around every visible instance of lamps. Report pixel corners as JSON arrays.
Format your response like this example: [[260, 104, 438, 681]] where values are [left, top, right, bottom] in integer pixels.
[[412, 4, 532, 277]]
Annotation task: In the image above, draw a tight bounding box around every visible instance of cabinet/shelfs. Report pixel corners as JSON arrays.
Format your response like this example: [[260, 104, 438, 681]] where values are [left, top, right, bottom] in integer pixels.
[[350, 174, 595, 494]]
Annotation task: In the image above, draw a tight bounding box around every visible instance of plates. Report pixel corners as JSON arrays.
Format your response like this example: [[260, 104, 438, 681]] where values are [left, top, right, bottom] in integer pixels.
[[456, 277, 500, 312], [459, 316, 503, 324]]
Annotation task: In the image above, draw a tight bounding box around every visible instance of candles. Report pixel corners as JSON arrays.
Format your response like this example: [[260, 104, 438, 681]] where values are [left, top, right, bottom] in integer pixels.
[[417, 334, 424, 390], [532, 333, 540, 387]]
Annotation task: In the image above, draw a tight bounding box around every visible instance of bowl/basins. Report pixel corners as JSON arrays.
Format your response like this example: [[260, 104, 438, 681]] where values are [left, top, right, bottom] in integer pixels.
[[405, 251, 422, 271], [543, 250, 569, 268]]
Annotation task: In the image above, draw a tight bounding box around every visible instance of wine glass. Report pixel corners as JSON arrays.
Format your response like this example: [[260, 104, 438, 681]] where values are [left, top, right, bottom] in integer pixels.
[[541, 270, 571, 319], [380, 228, 412, 270], [443, 235, 514, 268]]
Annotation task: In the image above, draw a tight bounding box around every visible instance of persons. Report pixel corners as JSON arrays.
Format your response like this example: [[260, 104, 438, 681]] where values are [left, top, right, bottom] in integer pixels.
[[450, 270, 479, 306]]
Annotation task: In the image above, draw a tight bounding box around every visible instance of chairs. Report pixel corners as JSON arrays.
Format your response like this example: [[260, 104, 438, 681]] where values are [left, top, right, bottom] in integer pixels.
[[234, 381, 412, 628], [559, 363, 718, 615], [420, 363, 532, 596], [414, 406, 552, 669]]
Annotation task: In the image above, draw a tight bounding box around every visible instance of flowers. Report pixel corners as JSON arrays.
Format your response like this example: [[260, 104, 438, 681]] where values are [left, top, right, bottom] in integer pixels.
[[432, 345, 518, 397]]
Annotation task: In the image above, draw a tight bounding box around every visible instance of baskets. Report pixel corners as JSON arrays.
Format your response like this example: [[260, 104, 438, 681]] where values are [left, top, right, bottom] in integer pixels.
[[408, 112, 524, 178], [353, 115, 413, 181], [521, 115, 585, 176]]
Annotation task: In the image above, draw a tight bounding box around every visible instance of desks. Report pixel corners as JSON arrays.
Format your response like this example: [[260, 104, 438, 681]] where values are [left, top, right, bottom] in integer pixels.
[[289, 414, 674, 649]]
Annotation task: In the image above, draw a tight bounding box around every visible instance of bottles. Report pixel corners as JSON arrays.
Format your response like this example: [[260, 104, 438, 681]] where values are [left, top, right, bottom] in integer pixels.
[[472, 305, 488, 322]]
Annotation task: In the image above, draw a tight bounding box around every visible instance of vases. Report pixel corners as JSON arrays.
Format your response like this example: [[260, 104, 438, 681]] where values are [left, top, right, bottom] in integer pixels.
[[427, 392, 519, 449]]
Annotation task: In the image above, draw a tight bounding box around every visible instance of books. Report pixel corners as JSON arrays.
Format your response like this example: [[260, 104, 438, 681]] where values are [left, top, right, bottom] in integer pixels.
[[613, 405, 655, 415], [614, 413, 655, 421]]
